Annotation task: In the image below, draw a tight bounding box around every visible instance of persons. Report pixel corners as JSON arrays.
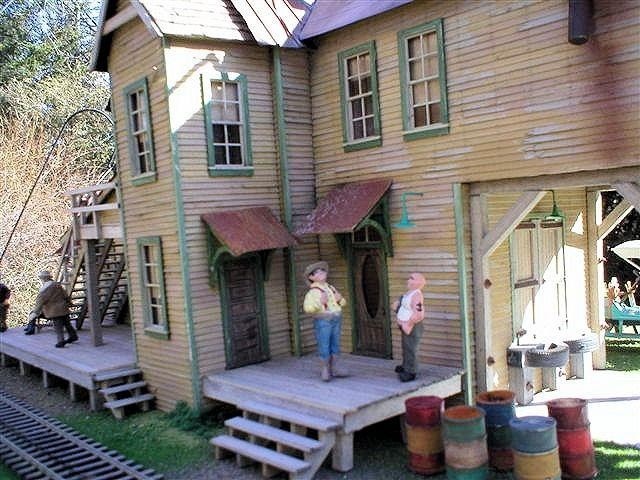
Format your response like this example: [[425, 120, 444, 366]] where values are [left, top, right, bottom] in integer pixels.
[[33, 270, 79, 348], [389, 272, 427, 382], [303, 260, 351, 381], [0, 280, 13, 332], [24, 310, 37, 334]]
